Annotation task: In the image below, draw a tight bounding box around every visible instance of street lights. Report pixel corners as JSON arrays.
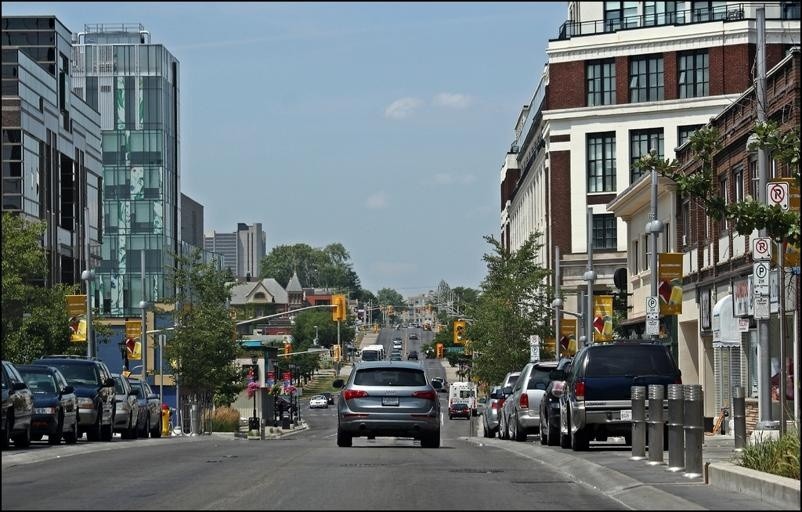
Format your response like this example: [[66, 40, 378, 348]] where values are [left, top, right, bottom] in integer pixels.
[[583, 270, 596, 347], [276, 348, 328, 357], [138, 300, 151, 382], [271, 358, 279, 427], [645, 219, 663, 341], [251, 354, 259, 429], [81, 270, 95, 358], [746, 125, 779, 430], [578, 336, 586, 349], [552, 298, 562, 362], [288, 362, 300, 424]]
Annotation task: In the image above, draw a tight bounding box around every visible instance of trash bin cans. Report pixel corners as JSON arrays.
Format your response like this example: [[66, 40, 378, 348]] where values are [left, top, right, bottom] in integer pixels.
[[248, 416, 259, 432], [281, 416, 291, 430]]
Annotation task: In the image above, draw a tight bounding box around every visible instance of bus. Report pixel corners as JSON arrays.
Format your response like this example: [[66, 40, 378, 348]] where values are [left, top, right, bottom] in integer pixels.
[[359, 344, 384, 363]]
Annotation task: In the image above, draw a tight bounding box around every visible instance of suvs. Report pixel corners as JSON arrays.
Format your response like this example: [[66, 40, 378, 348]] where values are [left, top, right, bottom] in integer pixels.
[[539, 340, 682, 450]]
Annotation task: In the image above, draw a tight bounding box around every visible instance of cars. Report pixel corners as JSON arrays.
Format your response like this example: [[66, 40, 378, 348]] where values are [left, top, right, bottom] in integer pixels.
[[409, 333, 417, 339], [333, 361, 440, 448], [310, 393, 334, 409], [406, 351, 419, 360], [390, 336, 402, 360], [0, 355, 162, 450], [431, 377, 449, 393], [448, 404, 471, 420], [479, 361, 560, 442]]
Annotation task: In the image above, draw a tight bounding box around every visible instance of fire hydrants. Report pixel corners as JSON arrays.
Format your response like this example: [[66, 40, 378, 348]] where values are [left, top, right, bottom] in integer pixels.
[[161, 403, 173, 437]]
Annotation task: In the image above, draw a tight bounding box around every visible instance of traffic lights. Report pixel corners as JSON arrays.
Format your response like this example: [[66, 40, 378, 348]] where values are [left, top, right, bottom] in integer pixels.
[[454, 321, 466, 344], [436, 343, 444, 358], [387, 303, 393, 317]]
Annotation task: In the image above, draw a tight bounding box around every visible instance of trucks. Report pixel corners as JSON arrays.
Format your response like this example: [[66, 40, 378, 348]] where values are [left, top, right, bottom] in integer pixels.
[[448, 380, 477, 417]]
